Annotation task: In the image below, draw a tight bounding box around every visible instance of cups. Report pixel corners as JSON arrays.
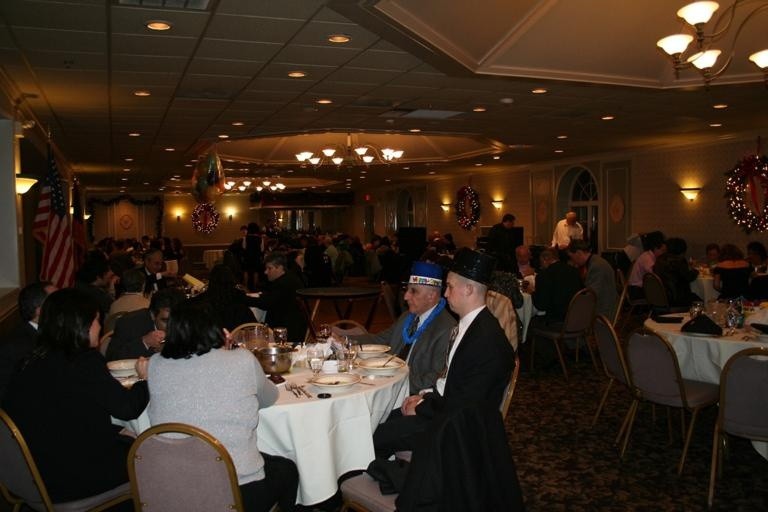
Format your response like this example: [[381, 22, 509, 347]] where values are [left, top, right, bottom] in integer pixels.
[[244, 324, 269, 351], [336, 350, 348, 373]]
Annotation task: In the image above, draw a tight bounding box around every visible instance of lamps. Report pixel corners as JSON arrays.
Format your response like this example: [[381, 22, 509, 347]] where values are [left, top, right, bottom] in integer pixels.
[[296, 133, 404, 173], [680, 188, 704, 202], [224, 177, 286, 195], [222, 204, 242, 223], [171, 206, 188, 222], [440, 203, 453, 213], [16, 177, 38, 194], [490, 200, 503, 209], [656, 0, 768, 84]]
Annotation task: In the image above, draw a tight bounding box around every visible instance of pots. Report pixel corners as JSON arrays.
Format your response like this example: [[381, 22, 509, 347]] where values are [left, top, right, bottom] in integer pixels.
[[253, 347, 292, 374]]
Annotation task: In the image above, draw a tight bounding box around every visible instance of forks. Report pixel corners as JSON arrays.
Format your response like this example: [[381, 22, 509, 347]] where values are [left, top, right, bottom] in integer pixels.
[[284, 383, 302, 398]]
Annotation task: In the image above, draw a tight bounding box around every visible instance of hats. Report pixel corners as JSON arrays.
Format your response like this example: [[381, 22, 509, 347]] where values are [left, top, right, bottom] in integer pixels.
[[450, 245, 496, 288], [409, 261, 443, 287]]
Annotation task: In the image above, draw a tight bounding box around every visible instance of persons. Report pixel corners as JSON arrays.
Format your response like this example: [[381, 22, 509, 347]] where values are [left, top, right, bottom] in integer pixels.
[[245, 252, 304, 343], [0, 287, 152, 512], [145, 299, 299, 512], [224, 217, 457, 285], [627, 229, 768, 312], [104, 286, 187, 362], [1, 281, 57, 397], [484, 210, 618, 369], [331, 260, 458, 398], [76, 235, 189, 335], [190, 264, 258, 332], [373, 245, 525, 512]]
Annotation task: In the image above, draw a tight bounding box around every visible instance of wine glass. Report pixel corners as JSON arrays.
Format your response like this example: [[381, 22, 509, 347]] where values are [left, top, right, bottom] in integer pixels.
[[273, 328, 288, 347], [688, 298, 742, 334], [320, 322, 332, 343], [343, 334, 358, 370], [307, 349, 324, 381]]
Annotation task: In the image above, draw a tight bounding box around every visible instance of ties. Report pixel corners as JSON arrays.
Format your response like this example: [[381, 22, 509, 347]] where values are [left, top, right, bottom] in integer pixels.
[[397, 316, 419, 361]]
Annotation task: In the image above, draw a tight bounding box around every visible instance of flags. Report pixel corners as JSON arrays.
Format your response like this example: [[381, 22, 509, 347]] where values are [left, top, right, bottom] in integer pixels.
[[31, 159, 76, 288]]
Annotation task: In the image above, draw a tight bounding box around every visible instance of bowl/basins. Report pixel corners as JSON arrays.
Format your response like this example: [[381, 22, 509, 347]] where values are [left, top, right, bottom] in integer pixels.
[[311, 373, 361, 391], [107, 359, 139, 377], [353, 344, 407, 377]]
